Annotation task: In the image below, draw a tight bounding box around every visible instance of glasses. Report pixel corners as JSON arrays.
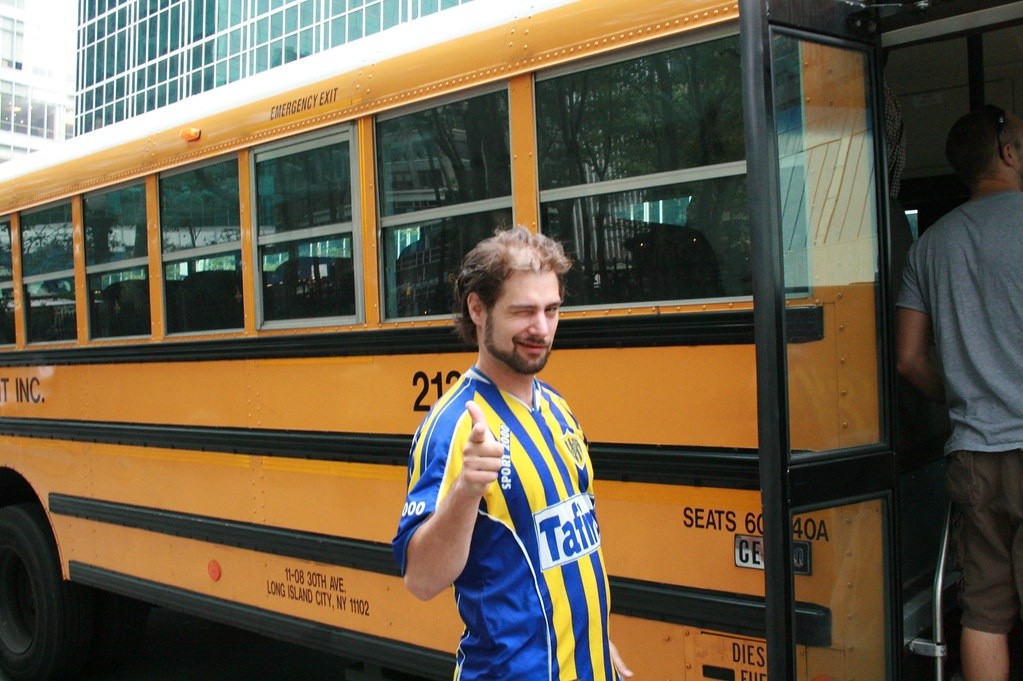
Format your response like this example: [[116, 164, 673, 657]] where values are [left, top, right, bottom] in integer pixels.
[[989, 103, 1007, 158]]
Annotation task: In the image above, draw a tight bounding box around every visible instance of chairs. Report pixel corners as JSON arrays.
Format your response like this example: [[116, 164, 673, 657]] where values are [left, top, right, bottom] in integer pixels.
[[94, 221, 721, 338]]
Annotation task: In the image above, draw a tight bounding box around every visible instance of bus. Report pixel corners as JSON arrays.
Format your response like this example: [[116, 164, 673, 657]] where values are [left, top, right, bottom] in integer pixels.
[[0, 1, 1023, 681]]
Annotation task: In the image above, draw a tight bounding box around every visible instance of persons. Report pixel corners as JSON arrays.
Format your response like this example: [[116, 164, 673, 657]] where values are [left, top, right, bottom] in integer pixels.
[[392, 228, 634, 681], [893, 106, 1023, 681]]
